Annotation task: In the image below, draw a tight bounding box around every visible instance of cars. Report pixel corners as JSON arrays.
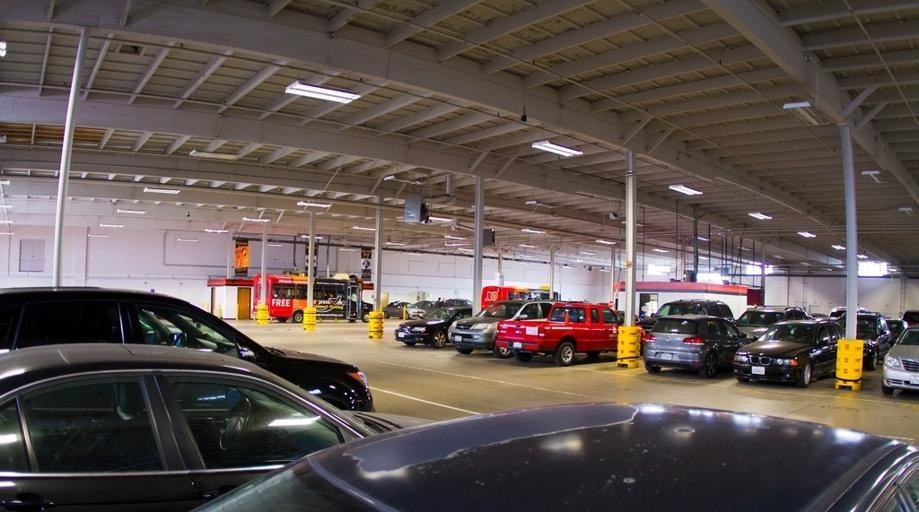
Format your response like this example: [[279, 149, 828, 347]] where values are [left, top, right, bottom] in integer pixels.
[[880, 325, 919, 395], [0, 341, 443, 511], [641, 314, 757, 376], [166, 396, 918, 512]]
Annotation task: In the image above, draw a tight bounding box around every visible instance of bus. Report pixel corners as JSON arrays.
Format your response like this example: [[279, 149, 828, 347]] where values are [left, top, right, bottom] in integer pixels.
[[253, 269, 375, 325], [480, 284, 561, 315]]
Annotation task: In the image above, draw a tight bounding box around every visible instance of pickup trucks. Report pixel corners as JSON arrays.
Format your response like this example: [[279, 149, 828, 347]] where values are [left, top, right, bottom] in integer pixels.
[[496, 299, 622, 367]]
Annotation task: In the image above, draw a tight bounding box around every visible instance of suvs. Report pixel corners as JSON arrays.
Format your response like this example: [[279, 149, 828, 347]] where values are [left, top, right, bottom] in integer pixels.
[[731, 318, 846, 388], [0, 282, 379, 414], [445, 298, 559, 358], [836, 310, 893, 370]]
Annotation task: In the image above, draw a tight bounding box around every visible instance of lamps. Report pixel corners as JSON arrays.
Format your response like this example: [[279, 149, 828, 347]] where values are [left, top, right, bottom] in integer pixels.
[[772, 252, 834, 281], [787, 101, 829, 131], [667, 182, 704, 200], [748, 211, 773, 223], [515, 194, 752, 277], [858, 254, 868, 260], [0, 143, 478, 253], [797, 230, 816, 241], [862, 167, 888, 187], [273, 77, 362, 112], [897, 205, 916, 217], [832, 243, 846, 252], [531, 139, 583, 162]]
